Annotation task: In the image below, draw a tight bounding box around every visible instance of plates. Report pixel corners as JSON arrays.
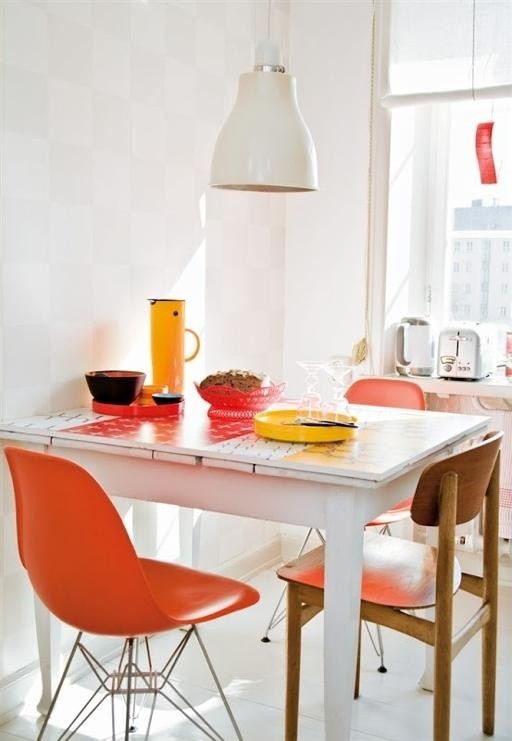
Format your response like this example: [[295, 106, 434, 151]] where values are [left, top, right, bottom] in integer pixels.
[[252, 408, 361, 444]]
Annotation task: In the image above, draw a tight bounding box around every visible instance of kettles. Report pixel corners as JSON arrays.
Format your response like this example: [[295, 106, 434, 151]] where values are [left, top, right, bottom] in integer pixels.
[[394, 314, 437, 377]]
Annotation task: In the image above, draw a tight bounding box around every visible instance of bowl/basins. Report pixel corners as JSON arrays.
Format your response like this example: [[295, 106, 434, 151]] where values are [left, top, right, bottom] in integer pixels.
[[84, 369, 146, 405], [151, 393, 185, 406]]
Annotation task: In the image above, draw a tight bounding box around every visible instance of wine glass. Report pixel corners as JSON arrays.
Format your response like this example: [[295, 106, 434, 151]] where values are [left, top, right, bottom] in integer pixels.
[[295, 359, 355, 415]]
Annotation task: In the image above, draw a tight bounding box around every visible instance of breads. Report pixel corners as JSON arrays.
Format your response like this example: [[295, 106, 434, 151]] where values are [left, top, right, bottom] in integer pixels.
[[199, 371, 262, 393]]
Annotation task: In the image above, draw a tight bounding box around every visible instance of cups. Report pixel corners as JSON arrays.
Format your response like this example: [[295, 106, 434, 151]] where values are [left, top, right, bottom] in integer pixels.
[[146, 298, 201, 394]]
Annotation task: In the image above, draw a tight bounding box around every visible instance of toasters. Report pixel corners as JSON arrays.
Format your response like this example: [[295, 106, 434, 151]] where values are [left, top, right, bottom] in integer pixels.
[[437, 320, 497, 381]]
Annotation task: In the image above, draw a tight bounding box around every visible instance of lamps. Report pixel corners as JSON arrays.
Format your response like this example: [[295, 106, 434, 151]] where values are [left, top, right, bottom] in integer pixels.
[[209, 0, 318, 193]]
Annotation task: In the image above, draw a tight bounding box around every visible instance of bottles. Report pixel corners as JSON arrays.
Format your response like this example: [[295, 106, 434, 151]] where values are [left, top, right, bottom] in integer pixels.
[[504, 331, 512, 378]]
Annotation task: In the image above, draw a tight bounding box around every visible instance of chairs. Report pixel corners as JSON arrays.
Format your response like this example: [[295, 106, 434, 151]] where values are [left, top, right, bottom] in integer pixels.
[[261, 378, 426, 673], [277, 430, 504, 741], [3, 446, 260, 741]]
